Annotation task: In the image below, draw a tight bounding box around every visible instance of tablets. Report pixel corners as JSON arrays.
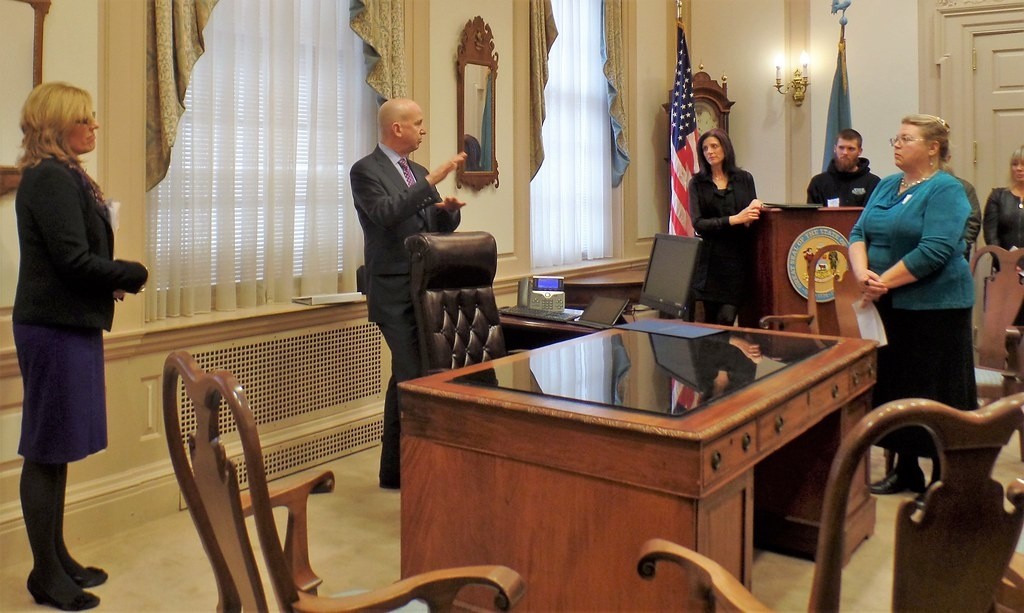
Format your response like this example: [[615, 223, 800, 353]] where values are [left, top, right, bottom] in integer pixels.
[[579, 295, 630, 326]]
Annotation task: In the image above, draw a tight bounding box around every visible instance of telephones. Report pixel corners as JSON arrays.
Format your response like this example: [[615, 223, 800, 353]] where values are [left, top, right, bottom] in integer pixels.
[[517, 276, 565, 312]]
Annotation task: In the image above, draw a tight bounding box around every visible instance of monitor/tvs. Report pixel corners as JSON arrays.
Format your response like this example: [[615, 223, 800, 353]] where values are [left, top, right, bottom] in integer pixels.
[[640, 233, 703, 319]]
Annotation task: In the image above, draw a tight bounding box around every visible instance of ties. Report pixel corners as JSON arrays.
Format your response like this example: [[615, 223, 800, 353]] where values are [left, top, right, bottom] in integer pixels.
[[398, 158, 416, 187]]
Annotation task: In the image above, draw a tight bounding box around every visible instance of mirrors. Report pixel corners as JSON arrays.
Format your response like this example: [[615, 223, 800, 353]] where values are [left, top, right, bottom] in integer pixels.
[[457, 15, 500, 190]]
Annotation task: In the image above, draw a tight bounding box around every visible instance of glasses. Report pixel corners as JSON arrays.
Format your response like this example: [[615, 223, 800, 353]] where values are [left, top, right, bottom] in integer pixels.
[[889, 136, 937, 147], [75, 111, 96, 124]]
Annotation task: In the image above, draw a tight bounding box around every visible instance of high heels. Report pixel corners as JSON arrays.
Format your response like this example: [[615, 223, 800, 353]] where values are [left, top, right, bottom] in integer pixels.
[[27, 569, 100, 611], [71, 566, 108, 588]]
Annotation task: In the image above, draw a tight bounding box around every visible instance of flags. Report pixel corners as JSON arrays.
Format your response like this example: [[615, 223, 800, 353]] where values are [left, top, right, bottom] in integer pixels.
[[668, 21, 701, 237], [822, 37, 852, 174], [672, 379, 701, 413]]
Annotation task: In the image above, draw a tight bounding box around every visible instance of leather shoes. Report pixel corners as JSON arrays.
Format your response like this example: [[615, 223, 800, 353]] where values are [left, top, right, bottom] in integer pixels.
[[871, 465, 925, 495], [914, 476, 940, 509]]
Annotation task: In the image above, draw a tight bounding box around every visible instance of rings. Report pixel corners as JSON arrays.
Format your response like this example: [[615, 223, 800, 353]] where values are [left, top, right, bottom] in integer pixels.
[[864, 277, 869, 285], [752, 353, 756, 356], [752, 210, 755, 213]]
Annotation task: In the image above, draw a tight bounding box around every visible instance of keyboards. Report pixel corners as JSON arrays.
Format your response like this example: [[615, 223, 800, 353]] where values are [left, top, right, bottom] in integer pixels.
[[501, 305, 580, 322]]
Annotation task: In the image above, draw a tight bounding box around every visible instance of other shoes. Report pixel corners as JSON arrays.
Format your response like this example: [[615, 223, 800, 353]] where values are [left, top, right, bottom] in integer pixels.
[[379, 473, 401, 489]]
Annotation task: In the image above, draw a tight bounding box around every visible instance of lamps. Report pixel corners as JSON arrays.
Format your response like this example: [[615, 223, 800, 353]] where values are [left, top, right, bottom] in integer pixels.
[[775, 48, 811, 110]]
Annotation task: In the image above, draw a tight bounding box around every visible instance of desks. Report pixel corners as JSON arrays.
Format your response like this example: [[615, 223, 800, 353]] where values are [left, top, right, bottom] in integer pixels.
[[397, 320, 880, 613], [494, 307, 597, 353]]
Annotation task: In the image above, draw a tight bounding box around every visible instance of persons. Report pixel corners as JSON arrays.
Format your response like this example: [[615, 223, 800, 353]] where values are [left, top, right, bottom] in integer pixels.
[[807, 129, 881, 207], [983, 145, 1024, 328], [847, 113, 979, 509], [11, 82, 149, 611], [697, 332, 764, 405], [939, 153, 982, 263], [350, 97, 467, 490], [688, 127, 763, 328]]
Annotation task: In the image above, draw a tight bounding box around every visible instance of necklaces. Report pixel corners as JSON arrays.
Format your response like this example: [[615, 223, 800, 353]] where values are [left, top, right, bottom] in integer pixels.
[[901, 176, 930, 188]]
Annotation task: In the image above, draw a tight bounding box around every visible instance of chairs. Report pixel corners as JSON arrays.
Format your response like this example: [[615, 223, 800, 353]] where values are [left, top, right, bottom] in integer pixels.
[[968, 246, 1024, 395], [636, 393, 1024, 612], [404, 230, 510, 374], [161, 352, 523, 612]]
